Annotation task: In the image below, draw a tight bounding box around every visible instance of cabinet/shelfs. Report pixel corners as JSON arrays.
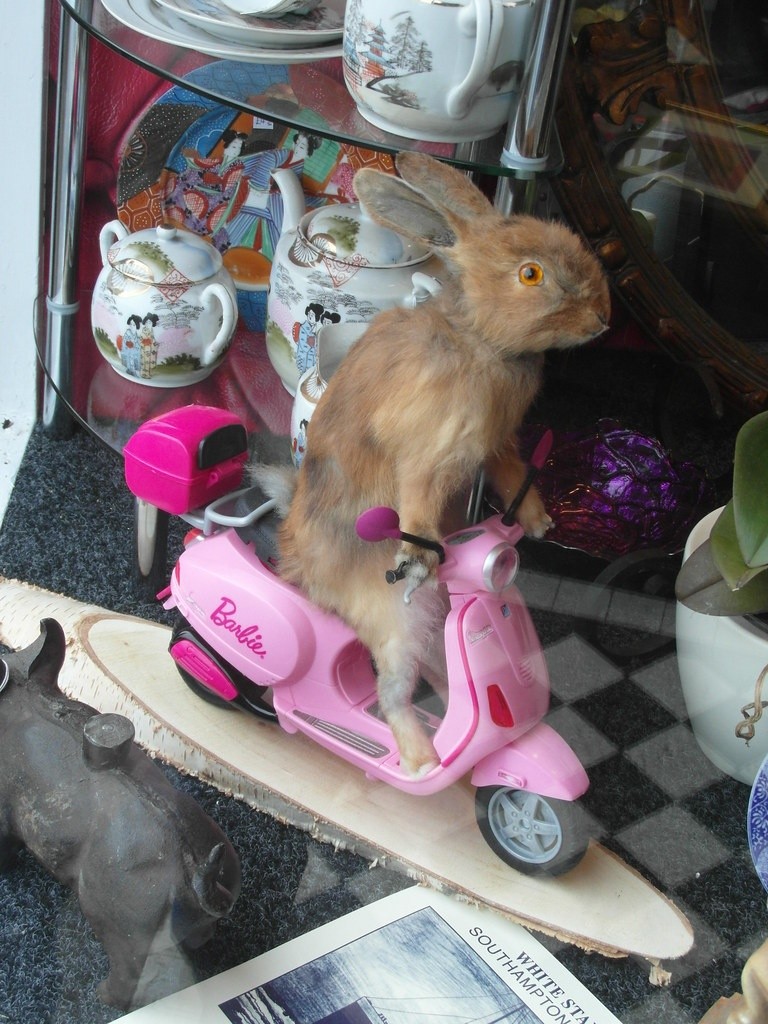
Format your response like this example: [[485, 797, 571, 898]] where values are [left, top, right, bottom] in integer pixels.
[[30, 0, 579, 582]]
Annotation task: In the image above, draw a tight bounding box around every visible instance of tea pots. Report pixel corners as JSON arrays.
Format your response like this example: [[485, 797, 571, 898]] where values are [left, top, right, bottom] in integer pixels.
[[270, 167, 450, 396], [344, 0, 539, 142]]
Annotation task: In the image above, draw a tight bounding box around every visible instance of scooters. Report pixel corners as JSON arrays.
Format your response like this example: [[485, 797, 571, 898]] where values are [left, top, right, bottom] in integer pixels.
[[123, 404, 593, 880]]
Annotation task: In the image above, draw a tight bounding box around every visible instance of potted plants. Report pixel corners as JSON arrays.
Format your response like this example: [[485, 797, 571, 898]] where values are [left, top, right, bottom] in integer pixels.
[[667, 403, 767, 790]]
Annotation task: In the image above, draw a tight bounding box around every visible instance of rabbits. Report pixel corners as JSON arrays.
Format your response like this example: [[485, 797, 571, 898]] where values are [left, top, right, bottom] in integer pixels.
[[240, 150, 609, 779]]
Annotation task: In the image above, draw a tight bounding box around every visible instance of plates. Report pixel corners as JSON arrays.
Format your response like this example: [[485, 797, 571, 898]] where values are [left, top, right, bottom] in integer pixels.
[[155, 1, 345, 46], [101, 0, 344, 64]]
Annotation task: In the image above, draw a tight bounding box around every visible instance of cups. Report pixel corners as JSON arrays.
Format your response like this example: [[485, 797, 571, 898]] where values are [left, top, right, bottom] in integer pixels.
[[90, 218, 237, 387], [289, 365, 332, 472]]
